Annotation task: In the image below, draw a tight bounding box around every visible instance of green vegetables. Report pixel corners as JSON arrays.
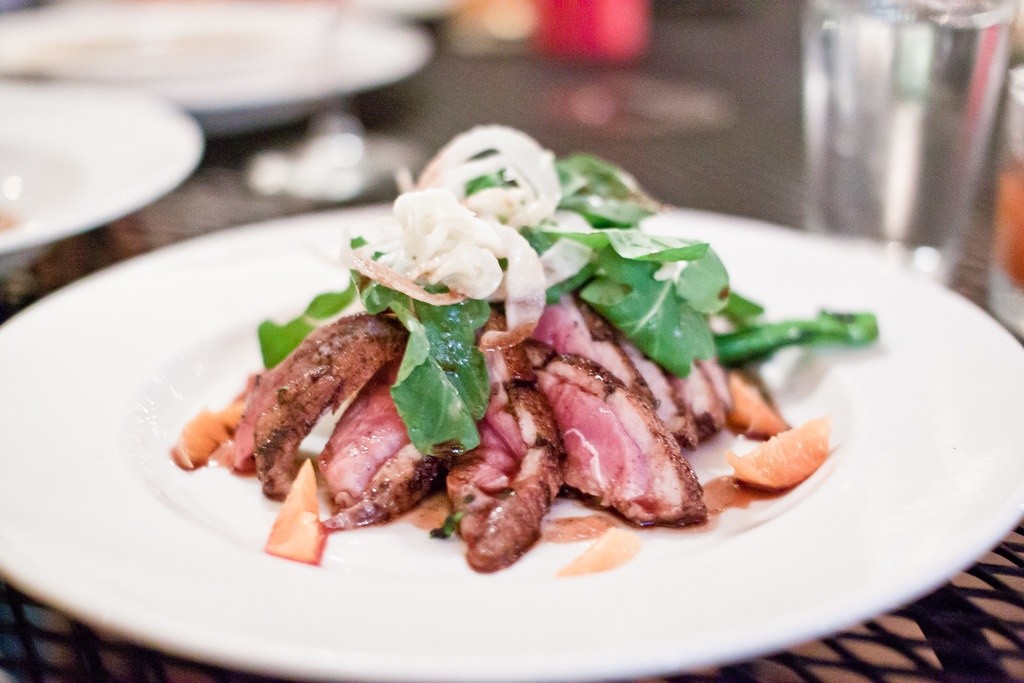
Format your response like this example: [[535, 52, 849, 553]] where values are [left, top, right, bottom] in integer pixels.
[[248, 153, 878, 458]]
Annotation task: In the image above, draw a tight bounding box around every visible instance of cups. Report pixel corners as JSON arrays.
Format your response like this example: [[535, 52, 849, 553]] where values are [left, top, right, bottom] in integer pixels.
[[797, 0, 1016, 313]]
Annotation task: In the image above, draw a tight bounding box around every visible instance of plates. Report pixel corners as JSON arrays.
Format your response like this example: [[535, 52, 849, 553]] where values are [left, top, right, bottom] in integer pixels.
[[0, 76, 207, 262], [0, 200, 1024, 683], [0, 0, 437, 139]]
